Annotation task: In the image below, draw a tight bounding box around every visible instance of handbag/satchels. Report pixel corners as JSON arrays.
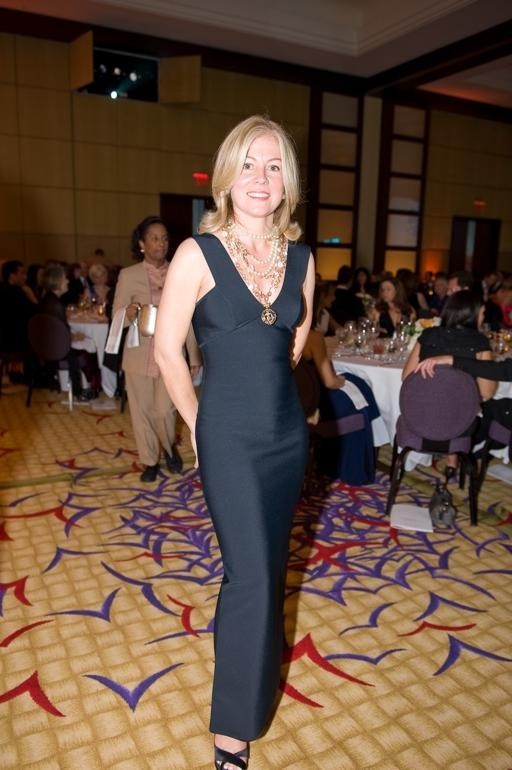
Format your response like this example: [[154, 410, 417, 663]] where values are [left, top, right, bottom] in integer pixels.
[[430, 488, 456, 526]]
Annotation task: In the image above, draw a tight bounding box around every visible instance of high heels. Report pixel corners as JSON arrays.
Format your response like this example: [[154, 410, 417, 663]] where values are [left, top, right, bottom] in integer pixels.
[[443, 466, 457, 487]]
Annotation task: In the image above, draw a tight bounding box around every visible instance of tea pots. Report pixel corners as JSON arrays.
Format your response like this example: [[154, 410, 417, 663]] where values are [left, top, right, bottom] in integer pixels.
[[128, 303, 158, 338]]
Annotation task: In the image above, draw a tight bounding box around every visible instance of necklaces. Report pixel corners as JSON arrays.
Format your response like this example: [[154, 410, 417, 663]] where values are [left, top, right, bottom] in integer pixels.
[[221, 219, 288, 326]]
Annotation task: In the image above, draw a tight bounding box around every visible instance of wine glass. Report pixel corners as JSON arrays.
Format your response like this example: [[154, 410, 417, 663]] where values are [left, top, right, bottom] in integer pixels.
[[482, 321, 511, 355]]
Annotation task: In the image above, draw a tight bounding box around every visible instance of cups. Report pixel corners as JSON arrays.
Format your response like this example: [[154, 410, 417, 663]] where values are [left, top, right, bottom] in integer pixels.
[[334, 319, 415, 365], [65, 296, 106, 321]]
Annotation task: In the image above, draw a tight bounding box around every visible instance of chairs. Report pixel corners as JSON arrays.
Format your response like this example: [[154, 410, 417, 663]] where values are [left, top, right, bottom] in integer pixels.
[[0, 267, 130, 414], [300, 281, 510, 529]]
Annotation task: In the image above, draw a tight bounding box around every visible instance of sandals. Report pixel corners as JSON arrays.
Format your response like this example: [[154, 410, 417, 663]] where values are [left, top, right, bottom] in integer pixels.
[[215, 746, 248, 770]]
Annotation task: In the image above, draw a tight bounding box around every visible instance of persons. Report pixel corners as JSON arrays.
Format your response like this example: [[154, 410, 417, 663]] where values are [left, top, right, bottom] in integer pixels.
[[152, 116, 318, 770], [2, 248, 129, 400], [290, 265, 512, 485], [111, 216, 202, 483]]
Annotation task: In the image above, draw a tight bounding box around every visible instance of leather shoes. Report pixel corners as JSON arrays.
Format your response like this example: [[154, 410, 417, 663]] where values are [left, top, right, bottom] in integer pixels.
[[141, 464, 160, 481], [165, 443, 183, 473]]
